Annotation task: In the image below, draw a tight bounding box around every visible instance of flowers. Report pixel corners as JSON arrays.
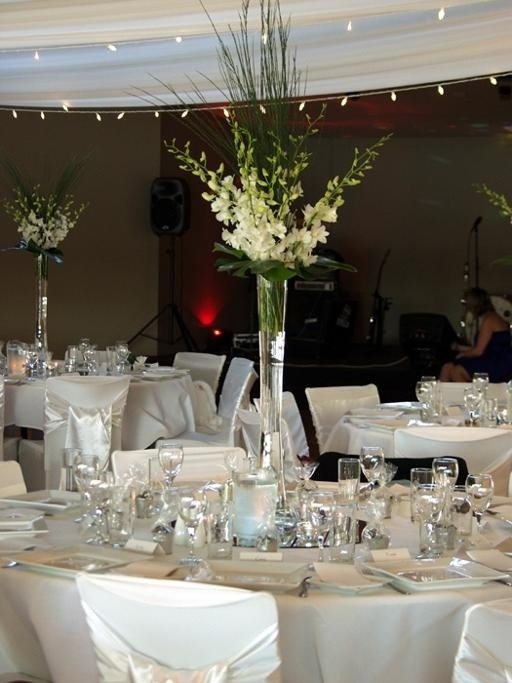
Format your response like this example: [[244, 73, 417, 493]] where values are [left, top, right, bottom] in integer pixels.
[[0, 147, 94, 349], [124, 0, 393, 432]]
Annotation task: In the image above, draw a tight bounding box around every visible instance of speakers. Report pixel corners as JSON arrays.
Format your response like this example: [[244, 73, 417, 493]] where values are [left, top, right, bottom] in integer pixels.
[[400, 313, 457, 360], [151, 176, 191, 235]]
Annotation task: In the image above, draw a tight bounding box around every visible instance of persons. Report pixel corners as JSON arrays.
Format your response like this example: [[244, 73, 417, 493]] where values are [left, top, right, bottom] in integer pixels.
[[438, 287, 512, 382]]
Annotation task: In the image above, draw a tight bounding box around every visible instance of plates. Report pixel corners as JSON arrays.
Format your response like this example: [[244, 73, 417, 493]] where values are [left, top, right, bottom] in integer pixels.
[[135, 362, 182, 380], [2, 487, 156, 576], [161, 560, 509, 593], [344, 396, 421, 429], [466, 496, 511, 586]]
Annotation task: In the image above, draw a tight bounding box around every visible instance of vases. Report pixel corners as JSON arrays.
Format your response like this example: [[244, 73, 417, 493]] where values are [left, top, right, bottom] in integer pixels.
[[257, 277, 287, 509], [32, 253, 48, 351]]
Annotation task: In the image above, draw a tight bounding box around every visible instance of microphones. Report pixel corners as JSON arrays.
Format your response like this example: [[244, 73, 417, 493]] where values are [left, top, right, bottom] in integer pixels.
[[471, 216, 482, 231]]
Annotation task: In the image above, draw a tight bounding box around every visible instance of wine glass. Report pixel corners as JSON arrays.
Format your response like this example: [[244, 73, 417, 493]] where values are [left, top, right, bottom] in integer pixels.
[[0, 337, 130, 381], [64, 445, 495, 562], [414, 372, 512, 428]]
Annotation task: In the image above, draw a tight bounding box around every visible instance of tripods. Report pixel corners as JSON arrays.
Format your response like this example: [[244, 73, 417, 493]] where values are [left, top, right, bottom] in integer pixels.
[[126, 235, 200, 351]]
